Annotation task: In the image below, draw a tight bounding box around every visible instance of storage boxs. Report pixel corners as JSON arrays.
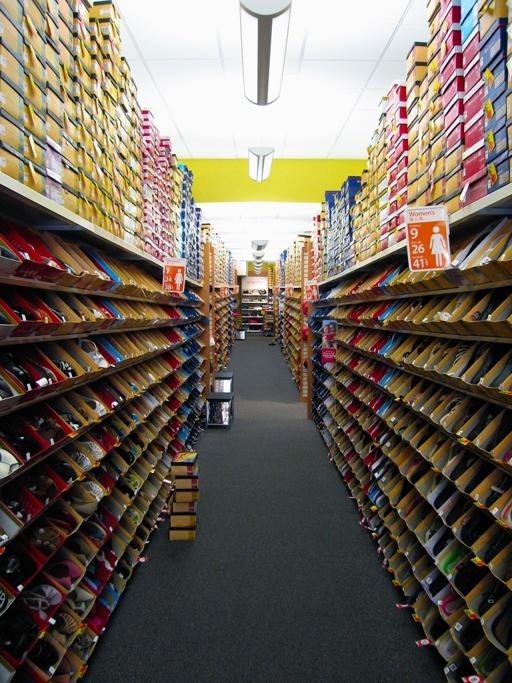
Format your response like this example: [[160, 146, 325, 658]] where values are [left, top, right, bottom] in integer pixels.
[[0, 1, 123, 683], [274, 39, 444, 646], [110, 49, 239, 587], [426, 1, 512, 683]]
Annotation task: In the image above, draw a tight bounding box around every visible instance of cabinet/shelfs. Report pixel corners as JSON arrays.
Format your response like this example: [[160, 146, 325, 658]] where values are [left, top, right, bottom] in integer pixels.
[[240, 288, 273, 334]]
